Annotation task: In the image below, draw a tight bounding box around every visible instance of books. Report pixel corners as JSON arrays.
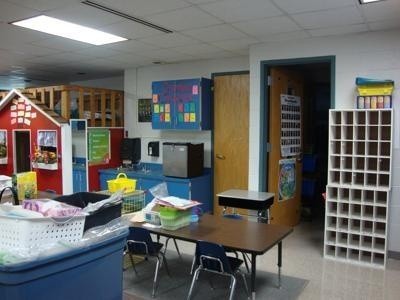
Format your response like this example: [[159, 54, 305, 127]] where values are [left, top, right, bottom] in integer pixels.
[[153, 194, 202, 211]]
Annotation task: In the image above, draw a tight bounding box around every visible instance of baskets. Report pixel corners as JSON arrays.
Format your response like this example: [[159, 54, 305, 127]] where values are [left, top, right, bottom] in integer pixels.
[[95, 189, 146, 215], [106, 173, 137, 194], [0, 197, 91, 260]]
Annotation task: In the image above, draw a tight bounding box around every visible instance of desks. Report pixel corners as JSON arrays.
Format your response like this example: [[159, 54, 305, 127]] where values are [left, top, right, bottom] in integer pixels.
[[132, 188, 294, 299]]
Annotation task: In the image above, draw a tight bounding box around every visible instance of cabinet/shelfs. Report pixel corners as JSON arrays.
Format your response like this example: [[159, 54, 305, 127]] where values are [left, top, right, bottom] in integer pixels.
[[151, 77, 213, 131], [99, 173, 211, 212], [322, 108, 394, 271]]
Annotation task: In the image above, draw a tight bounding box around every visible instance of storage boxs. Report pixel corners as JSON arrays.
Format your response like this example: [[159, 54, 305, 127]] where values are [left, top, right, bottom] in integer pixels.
[[0, 229, 129, 300]]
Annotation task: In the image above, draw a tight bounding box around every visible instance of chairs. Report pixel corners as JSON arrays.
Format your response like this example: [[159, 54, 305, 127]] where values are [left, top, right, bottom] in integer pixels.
[[123, 227, 171, 300]]
